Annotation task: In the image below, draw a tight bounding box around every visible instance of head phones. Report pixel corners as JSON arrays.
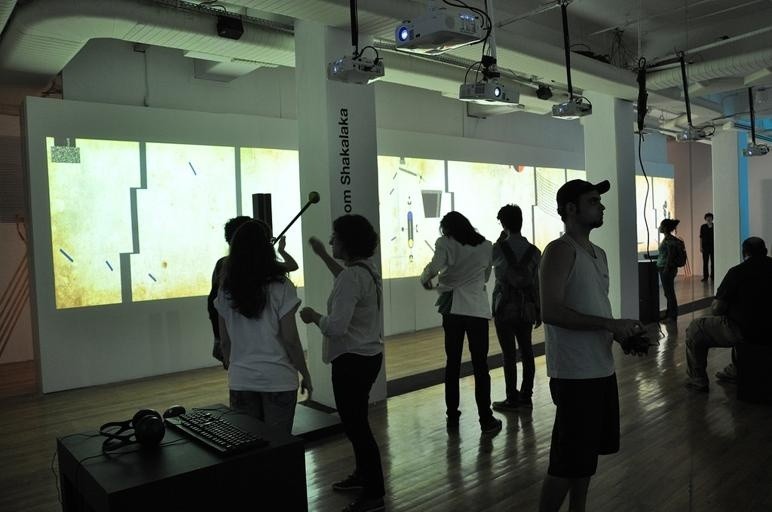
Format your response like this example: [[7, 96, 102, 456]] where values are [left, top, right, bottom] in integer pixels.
[[101, 409, 165, 450]]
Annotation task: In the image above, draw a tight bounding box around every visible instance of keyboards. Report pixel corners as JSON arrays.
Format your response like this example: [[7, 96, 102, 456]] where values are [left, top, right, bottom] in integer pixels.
[[166, 409, 264, 457]]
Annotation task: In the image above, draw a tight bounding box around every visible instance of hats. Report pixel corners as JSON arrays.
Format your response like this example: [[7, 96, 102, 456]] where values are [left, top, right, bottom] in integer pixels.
[[556, 179, 610, 205]]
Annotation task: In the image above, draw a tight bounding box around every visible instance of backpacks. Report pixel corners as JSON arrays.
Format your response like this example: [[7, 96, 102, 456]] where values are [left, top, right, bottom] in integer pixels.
[[495, 239, 537, 324], [665, 235, 686, 267]]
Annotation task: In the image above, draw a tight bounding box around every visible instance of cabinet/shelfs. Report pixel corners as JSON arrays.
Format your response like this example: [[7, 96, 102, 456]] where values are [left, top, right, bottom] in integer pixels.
[[56, 404, 308, 511]]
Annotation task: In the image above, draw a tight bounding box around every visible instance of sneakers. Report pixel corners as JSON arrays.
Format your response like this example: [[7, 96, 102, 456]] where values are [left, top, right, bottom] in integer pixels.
[[341, 497, 385, 512], [333, 476, 364, 490], [482, 420, 502, 434], [493, 398, 533, 412], [716, 363, 739, 381], [685, 369, 710, 391]]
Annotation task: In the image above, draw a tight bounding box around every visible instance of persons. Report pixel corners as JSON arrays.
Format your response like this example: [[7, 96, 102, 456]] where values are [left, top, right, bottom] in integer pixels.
[[644, 218, 680, 324], [698, 212, 714, 281], [205, 215, 253, 369], [299, 212, 387, 511], [421, 209, 504, 432], [534, 177, 652, 511], [486, 203, 544, 412], [212, 217, 315, 432], [680, 235, 772, 389]]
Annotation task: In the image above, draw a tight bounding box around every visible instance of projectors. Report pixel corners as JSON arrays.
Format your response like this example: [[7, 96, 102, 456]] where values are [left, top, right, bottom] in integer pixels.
[[328, 57, 384, 86], [741, 144, 769, 157], [552, 100, 592, 120], [674, 128, 706, 143], [394, 9, 482, 52], [459, 82, 519, 106]]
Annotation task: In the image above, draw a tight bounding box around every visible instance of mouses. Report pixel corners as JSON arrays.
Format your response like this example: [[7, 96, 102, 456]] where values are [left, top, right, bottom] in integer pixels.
[[162, 405, 185, 419]]
